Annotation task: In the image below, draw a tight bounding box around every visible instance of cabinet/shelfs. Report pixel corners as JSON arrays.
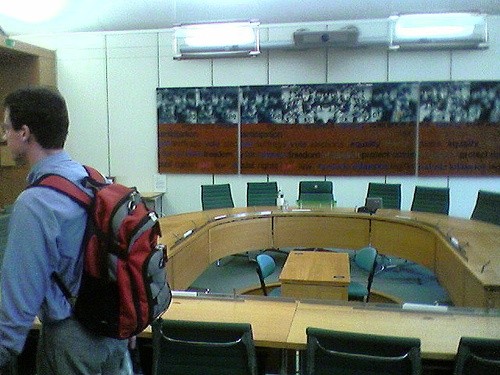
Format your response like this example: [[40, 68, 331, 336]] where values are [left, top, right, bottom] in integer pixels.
[[279, 250, 350, 303], [0, 35, 56, 208]]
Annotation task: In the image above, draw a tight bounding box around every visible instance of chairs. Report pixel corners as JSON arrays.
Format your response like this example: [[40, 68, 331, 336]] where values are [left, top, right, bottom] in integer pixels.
[[470, 190, 500, 225], [365, 183, 401, 263], [405, 186, 450, 263], [247, 182, 289, 262], [294, 181, 334, 252], [450, 337, 500, 375], [152, 319, 257, 375], [305, 327, 422, 375], [201, 184, 250, 267], [253, 252, 281, 297], [348, 247, 378, 303]]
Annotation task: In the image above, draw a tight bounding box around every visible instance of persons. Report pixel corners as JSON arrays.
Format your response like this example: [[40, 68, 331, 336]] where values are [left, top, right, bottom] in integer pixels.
[[0, 87, 136, 375]]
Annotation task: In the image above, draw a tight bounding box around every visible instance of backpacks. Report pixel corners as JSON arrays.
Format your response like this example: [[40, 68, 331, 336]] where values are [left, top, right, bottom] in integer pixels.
[[24, 165, 172, 340]]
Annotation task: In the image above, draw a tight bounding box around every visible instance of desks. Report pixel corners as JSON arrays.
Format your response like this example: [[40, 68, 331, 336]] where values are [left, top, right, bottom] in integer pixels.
[[141, 192, 164, 218], [134, 206, 500, 375]]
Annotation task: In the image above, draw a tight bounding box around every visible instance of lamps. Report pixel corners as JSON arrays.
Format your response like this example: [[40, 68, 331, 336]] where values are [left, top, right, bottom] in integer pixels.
[[178, 22, 256, 50], [294, 25, 359, 45], [393, 13, 486, 41]]
